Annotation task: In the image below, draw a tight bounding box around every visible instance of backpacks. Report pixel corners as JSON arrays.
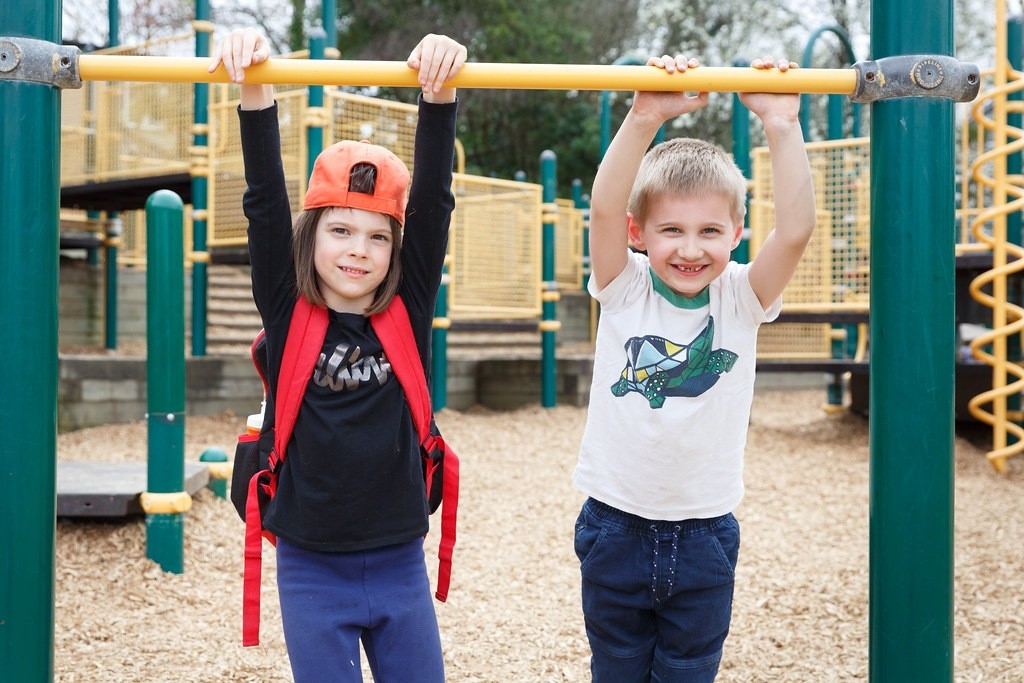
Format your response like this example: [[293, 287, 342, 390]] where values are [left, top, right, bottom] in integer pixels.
[[230, 288, 444, 546]]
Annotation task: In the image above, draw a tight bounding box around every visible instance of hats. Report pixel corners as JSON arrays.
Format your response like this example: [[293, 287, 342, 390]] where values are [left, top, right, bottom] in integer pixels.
[[302, 139, 411, 228]]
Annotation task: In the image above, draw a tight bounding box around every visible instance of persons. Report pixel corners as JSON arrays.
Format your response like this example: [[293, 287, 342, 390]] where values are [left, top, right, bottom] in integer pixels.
[[208, 27, 468, 683], [570, 54, 816, 683]]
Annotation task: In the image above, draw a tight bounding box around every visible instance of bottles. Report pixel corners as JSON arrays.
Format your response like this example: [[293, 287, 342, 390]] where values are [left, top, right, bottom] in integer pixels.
[[246, 401, 267, 436]]
